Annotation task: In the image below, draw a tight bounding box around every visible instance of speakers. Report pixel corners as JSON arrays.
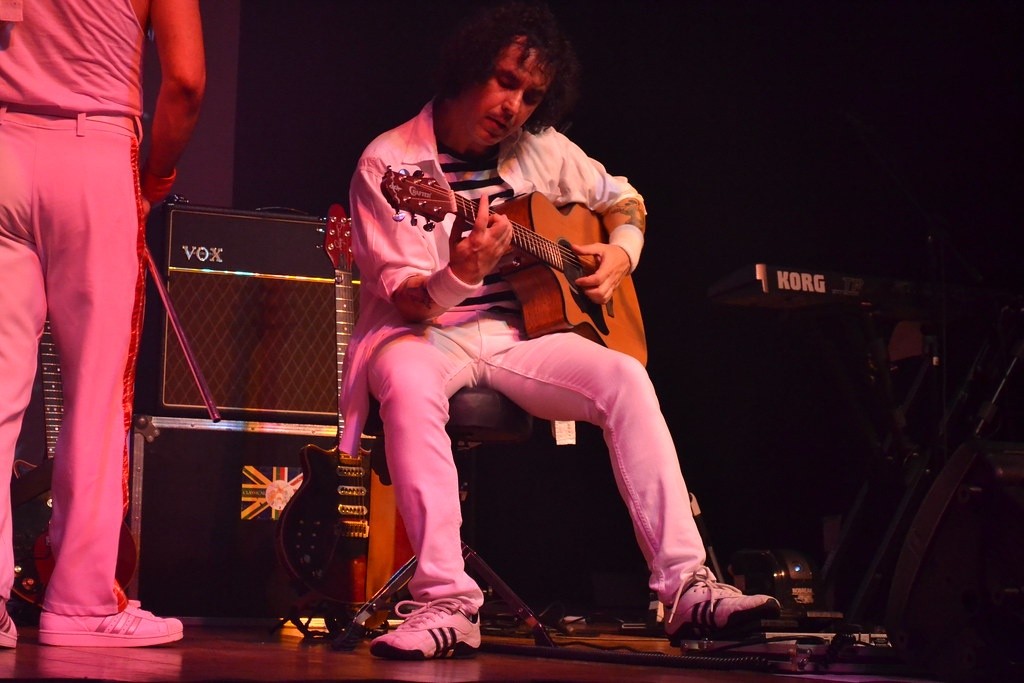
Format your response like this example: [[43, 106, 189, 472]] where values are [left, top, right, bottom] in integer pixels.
[[885, 441, 1022, 681]]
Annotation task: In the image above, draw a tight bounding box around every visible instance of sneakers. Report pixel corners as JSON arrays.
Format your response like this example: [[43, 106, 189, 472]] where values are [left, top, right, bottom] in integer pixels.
[[0, 600, 18, 649], [367, 603, 481, 661], [38, 600, 184, 645], [663, 579, 783, 655]]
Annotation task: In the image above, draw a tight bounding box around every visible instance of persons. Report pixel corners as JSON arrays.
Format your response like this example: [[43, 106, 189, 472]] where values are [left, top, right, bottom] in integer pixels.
[[0, 0, 205, 649], [333, 0, 784, 659]]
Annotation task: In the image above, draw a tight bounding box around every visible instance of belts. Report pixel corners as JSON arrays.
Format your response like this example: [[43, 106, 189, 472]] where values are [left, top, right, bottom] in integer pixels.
[[0, 100, 139, 136]]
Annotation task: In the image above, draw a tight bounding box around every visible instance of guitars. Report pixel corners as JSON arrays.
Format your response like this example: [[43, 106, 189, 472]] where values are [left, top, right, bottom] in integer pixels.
[[10, 300, 140, 611], [378, 163, 650, 371], [278, 204, 422, 606]]
